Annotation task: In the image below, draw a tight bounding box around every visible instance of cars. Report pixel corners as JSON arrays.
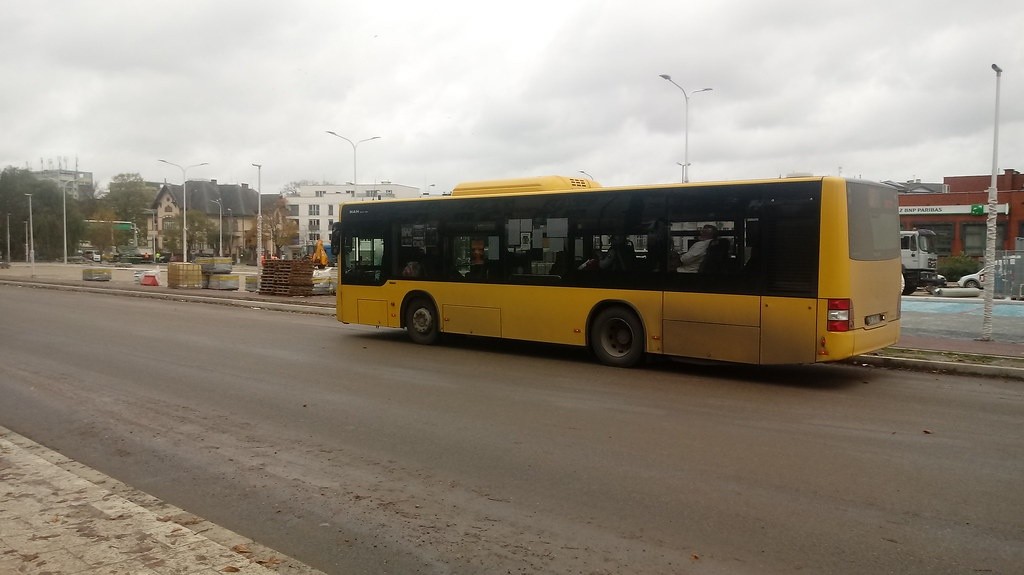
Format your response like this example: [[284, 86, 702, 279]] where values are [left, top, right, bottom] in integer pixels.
[[957, 258, 1018, 290]]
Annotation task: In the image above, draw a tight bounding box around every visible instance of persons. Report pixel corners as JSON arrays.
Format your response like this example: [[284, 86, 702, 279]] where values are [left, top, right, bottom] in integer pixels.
[[391, 218, 716, 282]]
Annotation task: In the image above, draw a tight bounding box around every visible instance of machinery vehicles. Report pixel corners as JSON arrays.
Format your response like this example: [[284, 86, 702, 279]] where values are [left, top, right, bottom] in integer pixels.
[[295, 237, 329, 268]]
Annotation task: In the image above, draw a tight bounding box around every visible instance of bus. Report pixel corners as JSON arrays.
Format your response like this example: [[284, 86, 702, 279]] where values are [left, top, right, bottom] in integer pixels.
[[328, 174, 904, 376]]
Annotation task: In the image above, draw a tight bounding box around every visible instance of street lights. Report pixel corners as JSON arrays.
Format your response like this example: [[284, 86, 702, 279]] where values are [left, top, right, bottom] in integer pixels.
[[50, 176, 84, 264], [208, 199, 227, 257], [21, 221, 31, 262], [249, 163, 267, 292], [144, 209, 159, 262], [24, 192, 41, 262], [658, 73, 714, 184], [5, 213, 15, 263], [326, 129, 382, 181], [157, 158, 211, 261], [974, 62, 1008, 344]]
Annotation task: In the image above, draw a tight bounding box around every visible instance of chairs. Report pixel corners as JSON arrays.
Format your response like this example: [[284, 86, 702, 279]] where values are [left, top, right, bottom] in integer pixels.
[[450, 238, 741, 284]]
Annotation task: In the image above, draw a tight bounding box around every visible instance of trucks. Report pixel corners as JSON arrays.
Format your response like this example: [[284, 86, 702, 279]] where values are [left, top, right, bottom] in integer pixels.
[[896, 229, 940, 298]]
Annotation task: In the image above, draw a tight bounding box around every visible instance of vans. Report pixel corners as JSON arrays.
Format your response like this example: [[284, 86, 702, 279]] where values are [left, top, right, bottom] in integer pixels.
[[84, 249, 105, 262]]
